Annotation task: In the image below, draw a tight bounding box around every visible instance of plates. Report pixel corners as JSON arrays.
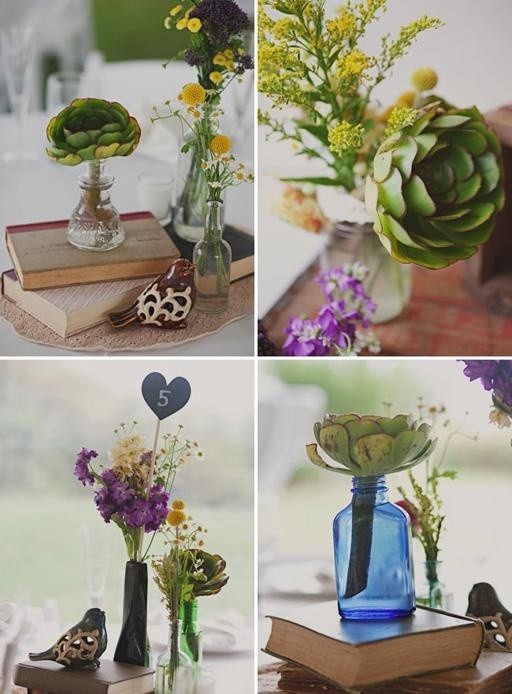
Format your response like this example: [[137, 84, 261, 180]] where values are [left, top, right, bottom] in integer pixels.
[[151, 626, 254, 656]]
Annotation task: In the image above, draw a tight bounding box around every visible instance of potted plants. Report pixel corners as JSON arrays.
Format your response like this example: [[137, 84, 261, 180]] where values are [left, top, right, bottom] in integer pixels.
[[47, 95, 147, 253], [305, 402, 437, 625], [169, 543, 227, 662]]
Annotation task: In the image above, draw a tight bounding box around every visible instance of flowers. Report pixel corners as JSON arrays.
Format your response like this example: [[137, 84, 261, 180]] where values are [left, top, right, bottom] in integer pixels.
[[258, 0, 441, 309], [132, 0, 253, 228], [194, 119, 255, 291], [455, 358, 511, 446], [133, 498, 204, 688], [72, 415, 203, 559], [378, 393, 482, 574], [278, 253, 391, 356]]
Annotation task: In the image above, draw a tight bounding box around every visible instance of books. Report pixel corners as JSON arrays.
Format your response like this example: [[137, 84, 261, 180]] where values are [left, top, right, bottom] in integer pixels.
[[260, 597, 512, 693], [12, 654, 157, 694], [2, 209, 255, 336]]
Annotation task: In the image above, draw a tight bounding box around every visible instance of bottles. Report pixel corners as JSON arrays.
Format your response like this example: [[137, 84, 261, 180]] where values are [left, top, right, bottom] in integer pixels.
[[328, 476, 425, 622]]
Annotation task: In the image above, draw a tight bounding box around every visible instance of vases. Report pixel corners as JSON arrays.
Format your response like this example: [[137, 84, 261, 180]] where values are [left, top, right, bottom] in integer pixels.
[[418, 558, 455, 613], [312, 207, 416, 325], [169, 140, 226, 240], [193, 198, 231, 314], [114, 556, 150, 667], [153, 623, 201, 693]]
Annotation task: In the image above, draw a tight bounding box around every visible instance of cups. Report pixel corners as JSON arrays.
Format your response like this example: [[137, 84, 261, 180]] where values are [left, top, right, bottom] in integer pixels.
[[136, 171, 175, 226], [44, 67, 100, 120]]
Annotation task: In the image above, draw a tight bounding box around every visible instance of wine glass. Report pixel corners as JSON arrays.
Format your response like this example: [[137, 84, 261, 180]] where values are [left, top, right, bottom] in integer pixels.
[[2, 24, 36, 152]]
